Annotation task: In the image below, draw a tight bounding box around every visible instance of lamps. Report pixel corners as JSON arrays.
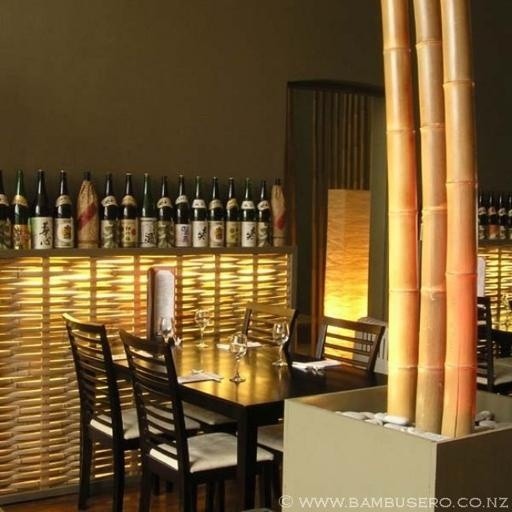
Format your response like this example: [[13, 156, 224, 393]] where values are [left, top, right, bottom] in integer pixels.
[[324, 189, 373, 362]]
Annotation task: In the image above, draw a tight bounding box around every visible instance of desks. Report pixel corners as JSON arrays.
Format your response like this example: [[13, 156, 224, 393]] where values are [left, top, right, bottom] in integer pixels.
[[104, 336, 389, 512]]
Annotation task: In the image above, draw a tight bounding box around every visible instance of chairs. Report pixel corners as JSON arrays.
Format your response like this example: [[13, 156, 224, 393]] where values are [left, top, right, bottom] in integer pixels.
[[236, 316, 385, 499], [119, 327, 275, 512], [164, 301, 299, 508], [475, 296, 511, 394], [63, 309, 199, 512]]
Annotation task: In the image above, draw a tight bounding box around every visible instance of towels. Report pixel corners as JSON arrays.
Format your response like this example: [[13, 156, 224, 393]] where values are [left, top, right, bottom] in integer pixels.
[[108, 349, 151, 361], [293, 355, 342, 373], [177, 364, 223, 386], [216, 339, 263, 350]]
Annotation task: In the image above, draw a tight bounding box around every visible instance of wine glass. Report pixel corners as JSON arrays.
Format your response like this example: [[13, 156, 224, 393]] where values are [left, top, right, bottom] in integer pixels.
[[194, 309, 211, 348], [273, 323, 289, 368], [158, 318, 173, 358], [230, 333, 247, 384]]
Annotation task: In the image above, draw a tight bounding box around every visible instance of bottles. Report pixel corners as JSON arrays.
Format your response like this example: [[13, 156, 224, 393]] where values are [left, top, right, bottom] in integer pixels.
[[0, 168, 285, 249], [476, 190, 512, 241]]
